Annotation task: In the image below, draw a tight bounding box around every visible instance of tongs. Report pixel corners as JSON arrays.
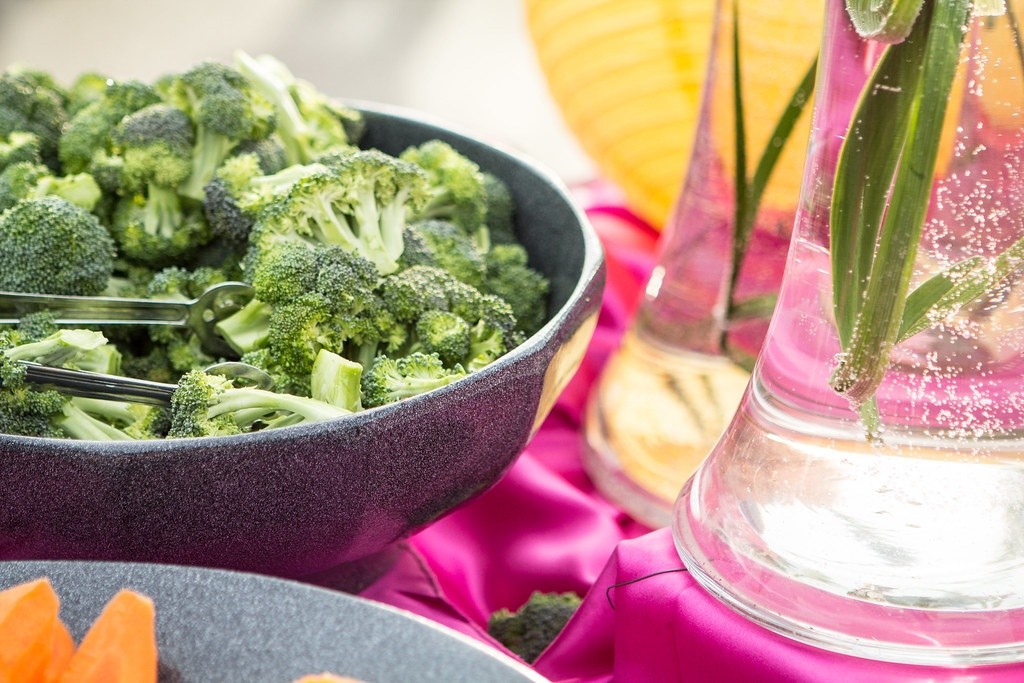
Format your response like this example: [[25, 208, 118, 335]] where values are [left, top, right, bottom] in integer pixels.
[[0, 280, 278, 407]]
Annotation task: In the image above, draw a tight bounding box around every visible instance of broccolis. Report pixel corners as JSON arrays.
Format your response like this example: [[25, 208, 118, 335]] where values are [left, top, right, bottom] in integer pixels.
[[484, 590, 583, 665], [0, 51, 548, 450]]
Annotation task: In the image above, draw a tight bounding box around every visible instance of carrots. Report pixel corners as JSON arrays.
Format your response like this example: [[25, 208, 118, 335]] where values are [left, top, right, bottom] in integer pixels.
[[0, 579, 366, 683]]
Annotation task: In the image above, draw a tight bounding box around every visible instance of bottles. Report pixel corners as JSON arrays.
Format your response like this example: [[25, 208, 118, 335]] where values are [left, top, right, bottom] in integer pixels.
[[671, 0, 1024, 670], [581, 0, 832, 527]]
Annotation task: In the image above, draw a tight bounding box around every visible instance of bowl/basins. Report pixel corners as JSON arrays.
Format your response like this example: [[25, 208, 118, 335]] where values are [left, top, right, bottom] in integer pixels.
[[0, 104, 608, 599], [0, 559, 550, 683]]
